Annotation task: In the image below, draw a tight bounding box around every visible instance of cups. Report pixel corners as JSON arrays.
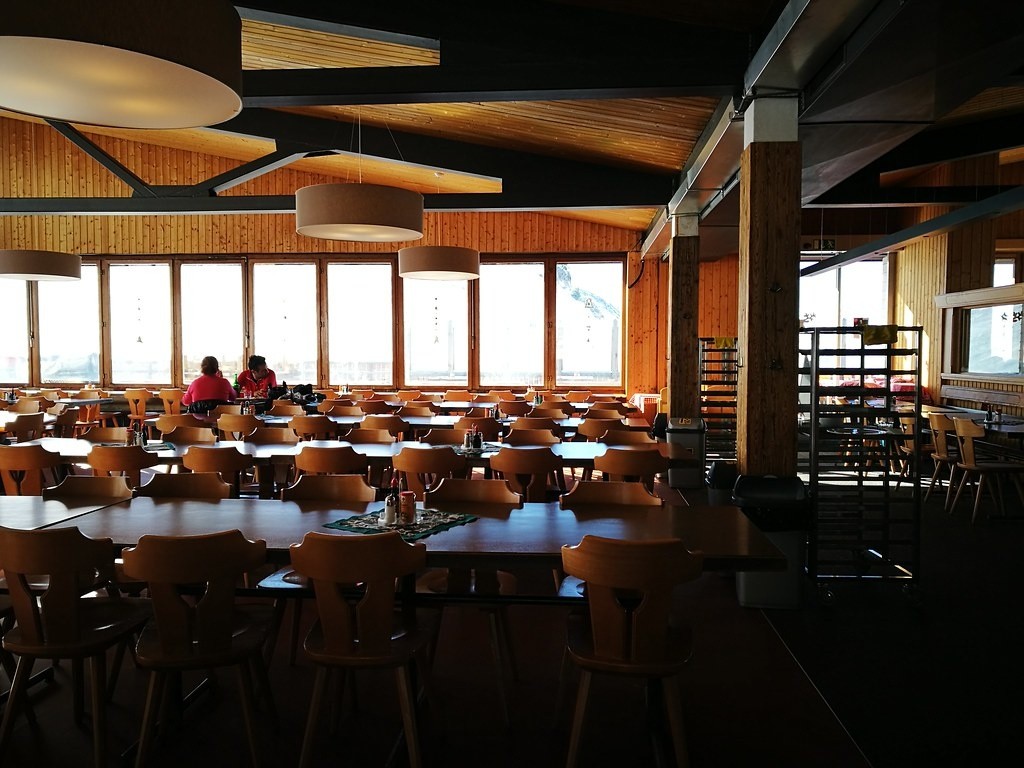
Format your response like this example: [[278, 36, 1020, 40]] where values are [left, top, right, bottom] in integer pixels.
[[240, 392, 244, 398]]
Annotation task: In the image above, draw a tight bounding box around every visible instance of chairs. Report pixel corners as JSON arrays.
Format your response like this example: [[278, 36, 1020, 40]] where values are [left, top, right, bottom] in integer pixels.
[[0, 382, 705, 768], [833, 382, 1024, 525]]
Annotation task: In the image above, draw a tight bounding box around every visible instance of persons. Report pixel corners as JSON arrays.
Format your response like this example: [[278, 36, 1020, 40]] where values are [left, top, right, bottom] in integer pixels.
[[238, 355, 277, 397], [181, 356, 236, 407]]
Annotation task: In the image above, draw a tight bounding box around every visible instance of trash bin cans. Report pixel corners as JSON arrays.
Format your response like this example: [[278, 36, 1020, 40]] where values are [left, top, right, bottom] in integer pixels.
[[653, 412, 667, 443], [728, 472, 810, 611], [707, 458, 738, 506], [665, 417, 706, 490]]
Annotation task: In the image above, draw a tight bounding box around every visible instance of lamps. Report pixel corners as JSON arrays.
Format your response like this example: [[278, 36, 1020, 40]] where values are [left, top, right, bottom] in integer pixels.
[[0, 248, 82, 281], [0, 1, 244, 131], [397, 245, 481, 282], [295, 105, 426, 242]]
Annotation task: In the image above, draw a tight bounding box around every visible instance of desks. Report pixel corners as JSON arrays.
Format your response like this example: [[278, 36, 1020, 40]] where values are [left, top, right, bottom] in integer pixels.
[[972, 415, 1023, 439], [916, 412, 1000, 425], [892, 403, 985, 419], [0, 386, 794, 738], [839, 397, 919, 410]]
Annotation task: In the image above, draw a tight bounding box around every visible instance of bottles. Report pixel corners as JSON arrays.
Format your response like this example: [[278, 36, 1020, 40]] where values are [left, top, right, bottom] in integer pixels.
[[473, 425, 481, 448], [387, 478, 399, 518], [495, 404, 499, 419], [233, 373, 240, 398]]
